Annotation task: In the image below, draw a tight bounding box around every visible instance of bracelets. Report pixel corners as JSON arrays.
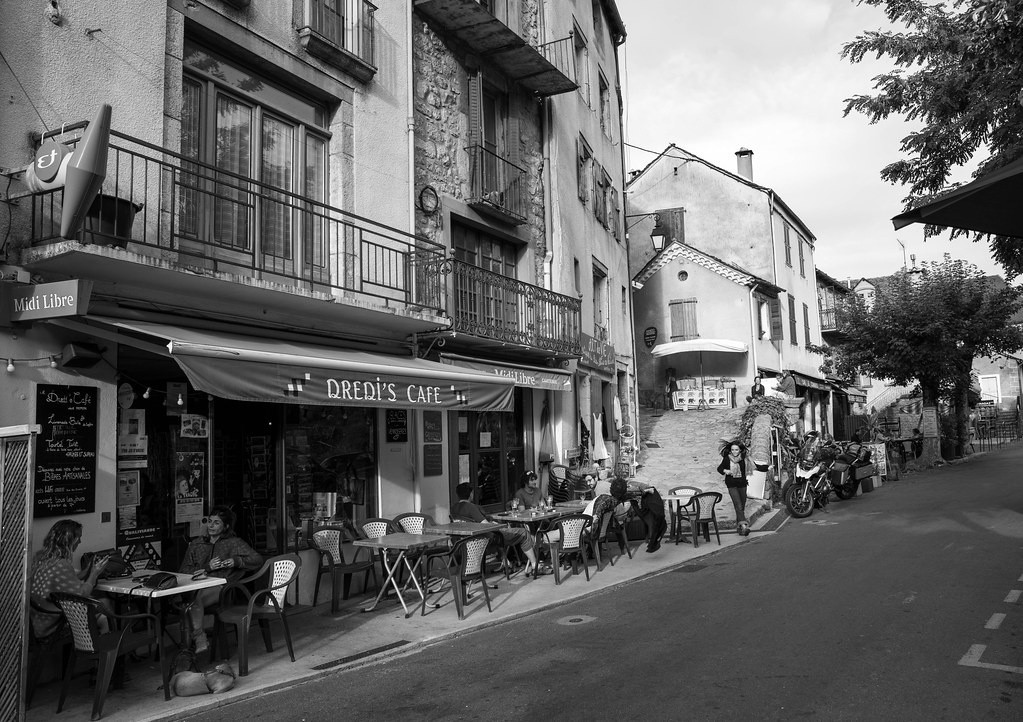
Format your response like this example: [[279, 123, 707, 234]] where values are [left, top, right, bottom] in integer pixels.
[[81, 581, 94, 588]]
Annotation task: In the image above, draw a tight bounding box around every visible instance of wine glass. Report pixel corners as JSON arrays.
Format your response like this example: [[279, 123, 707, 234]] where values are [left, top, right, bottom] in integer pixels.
[[511, 498, 519, 513], [580, 495, 585, 504], [546, 496, 553, 508]]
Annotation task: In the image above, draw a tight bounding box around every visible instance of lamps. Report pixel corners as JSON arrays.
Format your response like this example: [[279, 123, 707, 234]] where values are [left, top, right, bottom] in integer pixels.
[[62, 342, 102, 369], [625, 213, 666, 253]]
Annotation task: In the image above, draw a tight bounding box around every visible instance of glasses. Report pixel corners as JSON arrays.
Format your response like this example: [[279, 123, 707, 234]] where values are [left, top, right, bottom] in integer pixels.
[[526, 471, 534, 475]]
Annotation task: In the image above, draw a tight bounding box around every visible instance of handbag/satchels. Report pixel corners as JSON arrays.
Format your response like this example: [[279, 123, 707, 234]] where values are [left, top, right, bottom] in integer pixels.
[[143, 573, 178, 590], [81, 548, 132, 579]]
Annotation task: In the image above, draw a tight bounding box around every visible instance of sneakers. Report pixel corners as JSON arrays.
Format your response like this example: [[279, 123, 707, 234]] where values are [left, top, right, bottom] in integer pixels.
[[504, 564, 518, 575], [532, 566, 553, 576]]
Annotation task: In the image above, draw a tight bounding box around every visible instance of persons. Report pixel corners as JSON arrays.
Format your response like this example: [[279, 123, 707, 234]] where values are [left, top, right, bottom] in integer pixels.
[[717, 440, 775, 536], [177, 463, 201, 498], [667, 376, 678, 409], [583, 473, 612, 498], [190, 421, 202, 436], [28, 519, 124, 692], [771, 369, 796, 399], [851, 429, 862, 445], [452, 482, 552, 575], [516, 471, 542, 513], [180, 505, 263, 656], [910, 428, 923, 459], [541, 477, 628, 543], [746, 376, 764, 402]]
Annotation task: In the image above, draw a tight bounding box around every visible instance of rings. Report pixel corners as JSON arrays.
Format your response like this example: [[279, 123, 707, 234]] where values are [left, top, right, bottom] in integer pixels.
[[216, 562, 219, 564]]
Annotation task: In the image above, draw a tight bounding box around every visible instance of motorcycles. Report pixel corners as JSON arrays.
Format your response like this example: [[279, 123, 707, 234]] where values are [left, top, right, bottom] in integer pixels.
[[783, 431, 874, 519]]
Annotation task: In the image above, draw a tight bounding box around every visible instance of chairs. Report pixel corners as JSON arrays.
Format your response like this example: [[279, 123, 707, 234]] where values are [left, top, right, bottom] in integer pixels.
[[26, 537, 303, 721], [307, 464, 722, 620]]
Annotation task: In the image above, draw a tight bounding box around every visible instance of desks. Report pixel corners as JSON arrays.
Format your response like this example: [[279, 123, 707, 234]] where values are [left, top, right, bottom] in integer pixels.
[[487, 505, 585, 577], [94, 568, 227, 689], [352, 531, 451, 619], [892, 438, 918, 464], [672, 388, 737, 410], [555, 499, 596, 508], [422, 521, 507, 599], [658, 494, 692, 546]]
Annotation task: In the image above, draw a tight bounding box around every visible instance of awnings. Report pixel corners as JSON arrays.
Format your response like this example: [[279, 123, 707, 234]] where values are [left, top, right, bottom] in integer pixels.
[[39, 292, 515, 412], [427, 342, 575, 392], [758, 364, 831, 392], [827, 379, 866, 404]]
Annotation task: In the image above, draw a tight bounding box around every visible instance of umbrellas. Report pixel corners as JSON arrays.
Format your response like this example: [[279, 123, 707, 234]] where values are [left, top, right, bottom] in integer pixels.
[[650, 337, 749, 399], [537, 398, 555, 490]]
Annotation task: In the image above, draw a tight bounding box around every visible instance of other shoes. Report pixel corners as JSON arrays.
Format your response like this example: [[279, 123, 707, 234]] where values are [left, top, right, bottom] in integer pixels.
[[121, 671, 133, 684], [175, 649, 194, 673], [194, 633, 210, 654], [743, 528, 751, 536], [739, 528, 744, 536], [114, 674, 123, 688]]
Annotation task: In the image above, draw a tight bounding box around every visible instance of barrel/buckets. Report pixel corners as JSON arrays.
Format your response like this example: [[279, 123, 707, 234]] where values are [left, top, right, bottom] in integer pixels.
[[71, 191, 144, 250]]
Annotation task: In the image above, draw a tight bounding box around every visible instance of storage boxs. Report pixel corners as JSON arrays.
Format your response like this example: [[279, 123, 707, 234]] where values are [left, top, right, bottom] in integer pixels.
[[830, 442, 875, 485]]
